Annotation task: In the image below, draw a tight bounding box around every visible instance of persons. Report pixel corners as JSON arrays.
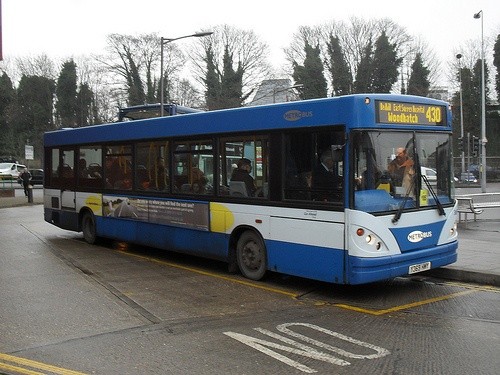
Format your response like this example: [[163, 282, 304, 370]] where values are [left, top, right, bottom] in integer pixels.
[[104, 157, 151, 189], [385, 144, 417, 199], [79, 159, 88, 176], [312, 150, 362, 202], [20, 168, 32, 197], [230, 158, 257, 197], [188, 176, 213, 194]]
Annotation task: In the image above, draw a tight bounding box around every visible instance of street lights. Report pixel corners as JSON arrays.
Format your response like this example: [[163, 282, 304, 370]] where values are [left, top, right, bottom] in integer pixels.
[[160, 30, 214, 116], [473, 10, 489, 193], [455, 52, 468, 187]]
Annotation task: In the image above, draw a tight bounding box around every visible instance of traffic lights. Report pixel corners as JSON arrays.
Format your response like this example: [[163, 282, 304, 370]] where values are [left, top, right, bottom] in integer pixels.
[[473, 137, 480, 151]]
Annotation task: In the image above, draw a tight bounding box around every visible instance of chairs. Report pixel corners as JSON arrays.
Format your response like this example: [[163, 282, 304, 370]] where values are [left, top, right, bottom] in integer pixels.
[[60, 155, 263, 200]]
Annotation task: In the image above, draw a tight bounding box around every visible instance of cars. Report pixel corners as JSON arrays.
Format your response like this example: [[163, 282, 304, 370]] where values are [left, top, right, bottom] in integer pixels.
[[0, 162, 27, 180], [462, 164, 480, 175], [418, 166, 459, 188], [464, 175, 478, 186], [18, 169, 44, 187]]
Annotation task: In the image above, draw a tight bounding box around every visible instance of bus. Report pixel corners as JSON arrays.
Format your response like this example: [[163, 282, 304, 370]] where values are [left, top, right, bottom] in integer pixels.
[[41, 92, 461, 284], [117, 104, 256, 185]]
